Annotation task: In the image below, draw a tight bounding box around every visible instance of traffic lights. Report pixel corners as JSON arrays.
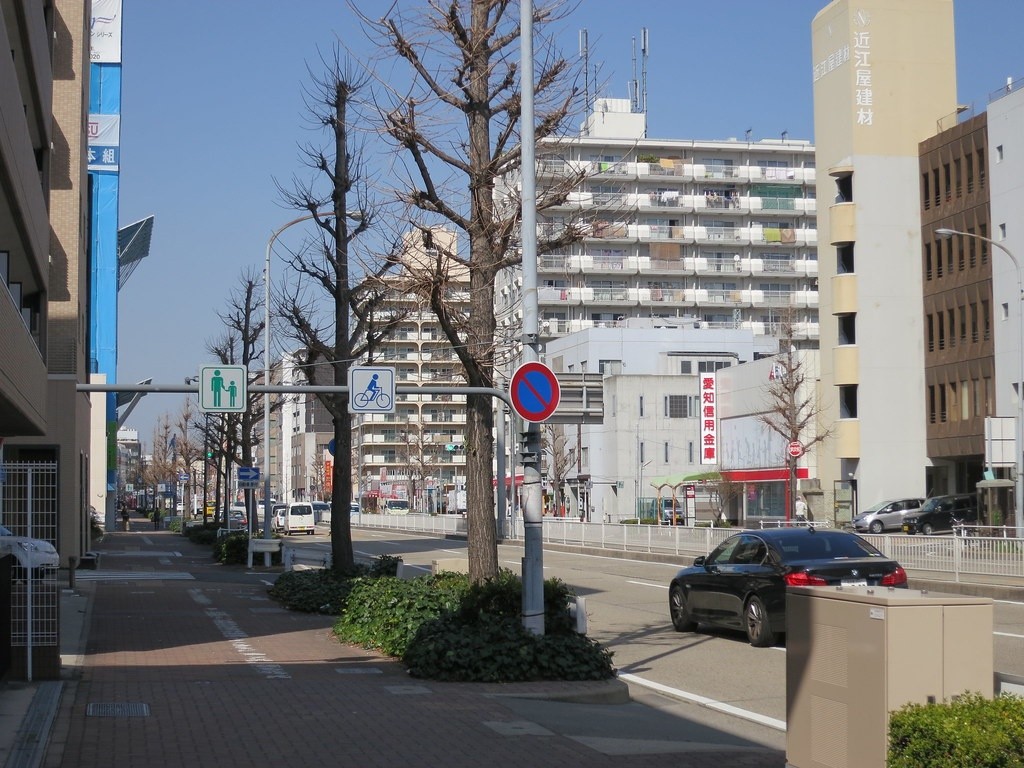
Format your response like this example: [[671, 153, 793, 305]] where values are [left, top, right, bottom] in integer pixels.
[[208, 452, 212, 457], [445, 444, 464, 451]]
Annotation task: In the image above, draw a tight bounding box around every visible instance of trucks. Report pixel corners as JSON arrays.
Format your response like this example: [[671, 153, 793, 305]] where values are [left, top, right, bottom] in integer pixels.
[[285, 502, 315, 536]]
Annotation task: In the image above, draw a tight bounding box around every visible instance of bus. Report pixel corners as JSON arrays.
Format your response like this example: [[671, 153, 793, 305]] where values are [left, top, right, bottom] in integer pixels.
[[201, 500, 217, 518]]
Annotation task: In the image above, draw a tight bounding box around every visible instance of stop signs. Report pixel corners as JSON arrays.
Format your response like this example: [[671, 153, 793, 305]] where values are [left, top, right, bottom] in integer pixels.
[[788, 442, 802, 457]]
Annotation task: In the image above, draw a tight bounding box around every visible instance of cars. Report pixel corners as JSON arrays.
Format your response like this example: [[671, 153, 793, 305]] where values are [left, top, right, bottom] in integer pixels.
[[176, 503, 186, 512], [900, 493, 985, 534], [312, 503, 330, 510], [257, 499, 276, 508], [649, 497, 684, 525], [270, 503, 287, 517], [385, 499, 409, 515], [271, 509, 286, 533], [852, 498, 927, 534], [351, 506, 360, 514], [669, 528, 908, 648], [0, 524, 60, 584], [220, 510, 246, 524]]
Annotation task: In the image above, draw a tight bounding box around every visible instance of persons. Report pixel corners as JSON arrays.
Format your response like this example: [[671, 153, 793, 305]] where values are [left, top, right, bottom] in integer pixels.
[[796, 496, 808, 521], [365, 503, 372, 513], [154, 508, 160, 530]]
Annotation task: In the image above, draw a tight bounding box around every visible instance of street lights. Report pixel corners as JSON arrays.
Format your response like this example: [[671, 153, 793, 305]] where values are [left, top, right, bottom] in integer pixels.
[[263, 210, 368, 566], [935, 228, 1023, 554]]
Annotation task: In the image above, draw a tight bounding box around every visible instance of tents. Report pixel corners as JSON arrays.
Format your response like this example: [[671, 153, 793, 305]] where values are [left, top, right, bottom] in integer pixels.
[[363, 490, 379, 510], [493, 475, 523, 517]]
